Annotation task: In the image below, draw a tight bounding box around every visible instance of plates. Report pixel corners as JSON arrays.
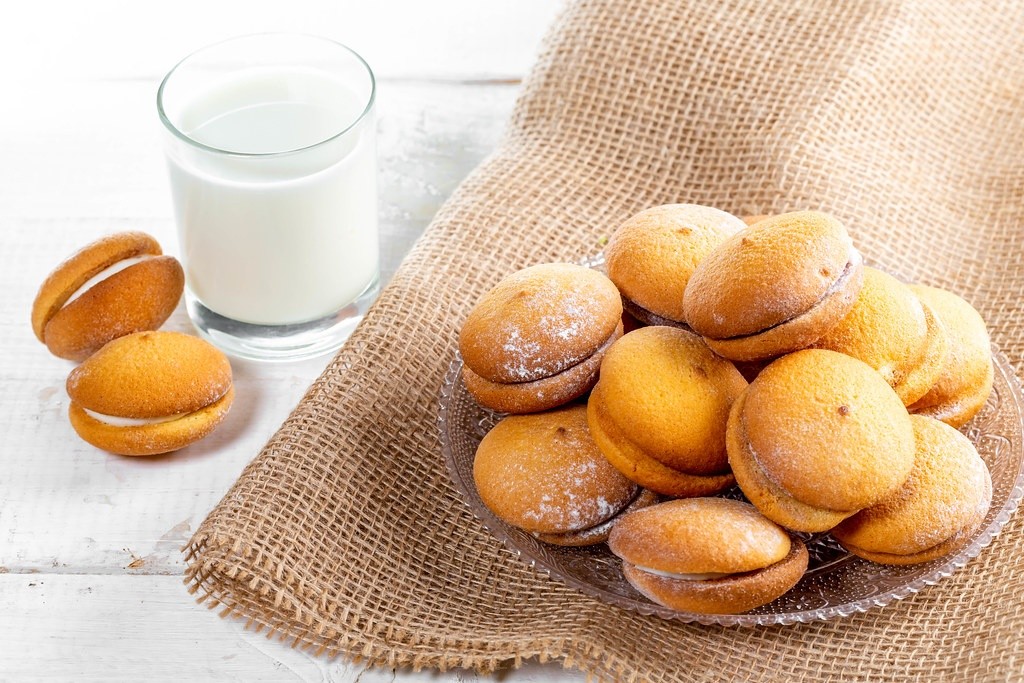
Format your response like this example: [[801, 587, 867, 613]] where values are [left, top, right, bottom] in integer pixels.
[[441, 260, 1024, 628]]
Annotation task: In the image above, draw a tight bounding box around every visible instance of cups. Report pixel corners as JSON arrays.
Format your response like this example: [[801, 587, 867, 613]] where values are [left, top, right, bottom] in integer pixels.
[[158, 36, 379, 363]]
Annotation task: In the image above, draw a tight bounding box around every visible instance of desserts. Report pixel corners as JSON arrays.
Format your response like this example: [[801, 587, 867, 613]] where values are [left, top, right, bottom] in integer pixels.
[[32, 230, 184, 360], [458, 203, 996, 612], [66, 328, 233, 455]]
